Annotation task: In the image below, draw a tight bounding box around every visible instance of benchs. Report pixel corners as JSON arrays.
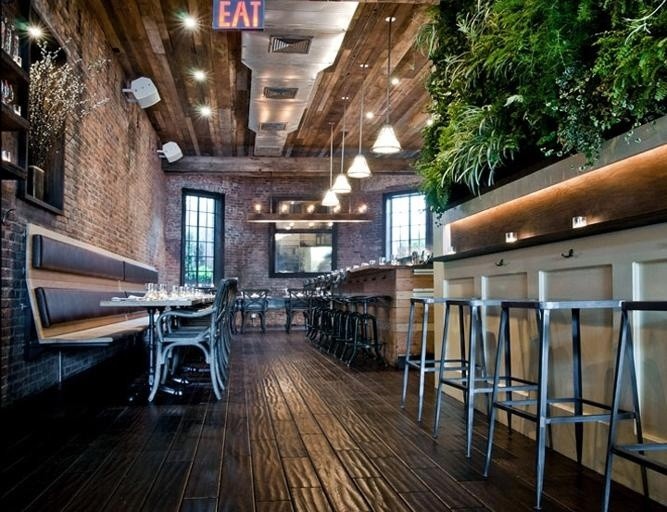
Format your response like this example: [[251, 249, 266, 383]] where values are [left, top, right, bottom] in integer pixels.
[[23, 222, 158, 390]]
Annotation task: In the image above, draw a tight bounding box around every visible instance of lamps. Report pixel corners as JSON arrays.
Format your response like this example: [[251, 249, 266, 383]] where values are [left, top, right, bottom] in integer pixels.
[[321, 15, 402, 206], [245, 204, 372, 222]]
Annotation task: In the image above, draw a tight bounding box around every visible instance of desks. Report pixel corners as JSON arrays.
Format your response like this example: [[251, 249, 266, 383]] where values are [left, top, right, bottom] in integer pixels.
[[100, 295, 217, 405], [284, 298, 307, 329]]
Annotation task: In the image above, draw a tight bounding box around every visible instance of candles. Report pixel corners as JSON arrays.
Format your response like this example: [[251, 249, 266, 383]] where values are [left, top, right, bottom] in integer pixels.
[[571, 216, 586, 228]]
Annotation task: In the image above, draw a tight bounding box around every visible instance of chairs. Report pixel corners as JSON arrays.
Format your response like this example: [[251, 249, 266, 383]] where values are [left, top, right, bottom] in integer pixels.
[[287, 289, 307, 334], [148, 278, 270, 401], [308, 273, 392, 368]]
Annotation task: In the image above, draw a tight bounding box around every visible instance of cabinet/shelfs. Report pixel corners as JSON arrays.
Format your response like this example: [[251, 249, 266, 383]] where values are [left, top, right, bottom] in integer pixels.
[[0, 48, 29, 179]]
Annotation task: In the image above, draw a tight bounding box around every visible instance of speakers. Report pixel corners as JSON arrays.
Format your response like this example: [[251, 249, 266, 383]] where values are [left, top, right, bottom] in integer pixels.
[[161, 141, 184, 164], [129, 75, 162, 110]]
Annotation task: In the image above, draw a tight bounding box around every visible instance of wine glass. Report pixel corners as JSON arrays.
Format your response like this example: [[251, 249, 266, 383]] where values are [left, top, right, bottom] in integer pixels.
[[143, 283, 205, 301]]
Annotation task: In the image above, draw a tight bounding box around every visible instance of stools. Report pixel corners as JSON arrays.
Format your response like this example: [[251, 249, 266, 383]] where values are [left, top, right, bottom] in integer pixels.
[[595, 299, 667, 512], [432, 299, 556, 458], [400, 297, 492, 424], [483, 296, 652, 511]]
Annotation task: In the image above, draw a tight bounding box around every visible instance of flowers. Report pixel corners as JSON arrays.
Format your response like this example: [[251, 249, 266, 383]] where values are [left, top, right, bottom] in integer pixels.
[[29, 37, 112, 165]]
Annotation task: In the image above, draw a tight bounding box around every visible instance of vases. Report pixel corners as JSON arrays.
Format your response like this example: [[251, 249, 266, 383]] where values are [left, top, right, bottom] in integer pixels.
[[28, 165, 45, 201]]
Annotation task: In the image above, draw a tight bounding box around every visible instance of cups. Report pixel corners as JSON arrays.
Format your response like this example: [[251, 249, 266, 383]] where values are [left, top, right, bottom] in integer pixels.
[[317, 255, 397, 280], [573, 217, 587, 228]]
